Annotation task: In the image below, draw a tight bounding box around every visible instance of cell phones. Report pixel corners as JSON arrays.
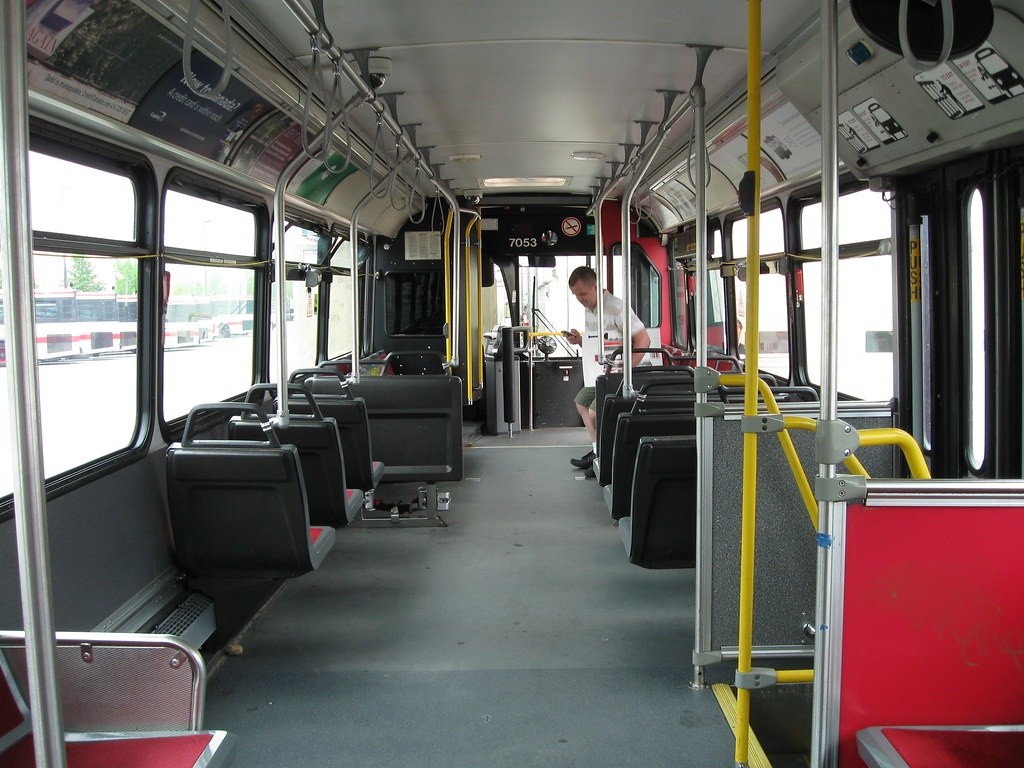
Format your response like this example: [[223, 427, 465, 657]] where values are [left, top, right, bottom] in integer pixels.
[[561, 331, 577, 338]]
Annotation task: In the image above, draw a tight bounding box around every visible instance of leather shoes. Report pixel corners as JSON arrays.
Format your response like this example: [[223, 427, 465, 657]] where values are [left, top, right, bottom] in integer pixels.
[[584, 469, 596, 477], [570, 450, 596, 468]]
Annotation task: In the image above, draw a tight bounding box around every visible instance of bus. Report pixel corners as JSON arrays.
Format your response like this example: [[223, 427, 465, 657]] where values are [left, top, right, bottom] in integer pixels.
[[0, 288, 81, 368], [77, 291, 121, 356], [115, 294, 276, 354]]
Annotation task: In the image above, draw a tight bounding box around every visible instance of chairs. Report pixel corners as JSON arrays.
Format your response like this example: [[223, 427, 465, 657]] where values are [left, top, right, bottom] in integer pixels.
[[163, 405, 335, 580], [290, 368, 384, 489], [231, 381, 362, 527], [378, 353, 451, 379]]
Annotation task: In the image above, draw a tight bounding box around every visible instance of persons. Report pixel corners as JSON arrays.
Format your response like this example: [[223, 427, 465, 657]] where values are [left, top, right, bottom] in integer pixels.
[[563, 266, 651, 477], [738, 319, 745, 353]]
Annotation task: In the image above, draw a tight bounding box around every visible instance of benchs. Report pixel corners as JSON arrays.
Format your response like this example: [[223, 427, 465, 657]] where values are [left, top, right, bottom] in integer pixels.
[[617, 437, 698, 571], [287, 361, 466, 532], [604, 384, 818, 519], [0, 628, 227, 768], [855, 726, 1024, 768], [592, 372, 774, 487], [607, 350, 740, 429]]
